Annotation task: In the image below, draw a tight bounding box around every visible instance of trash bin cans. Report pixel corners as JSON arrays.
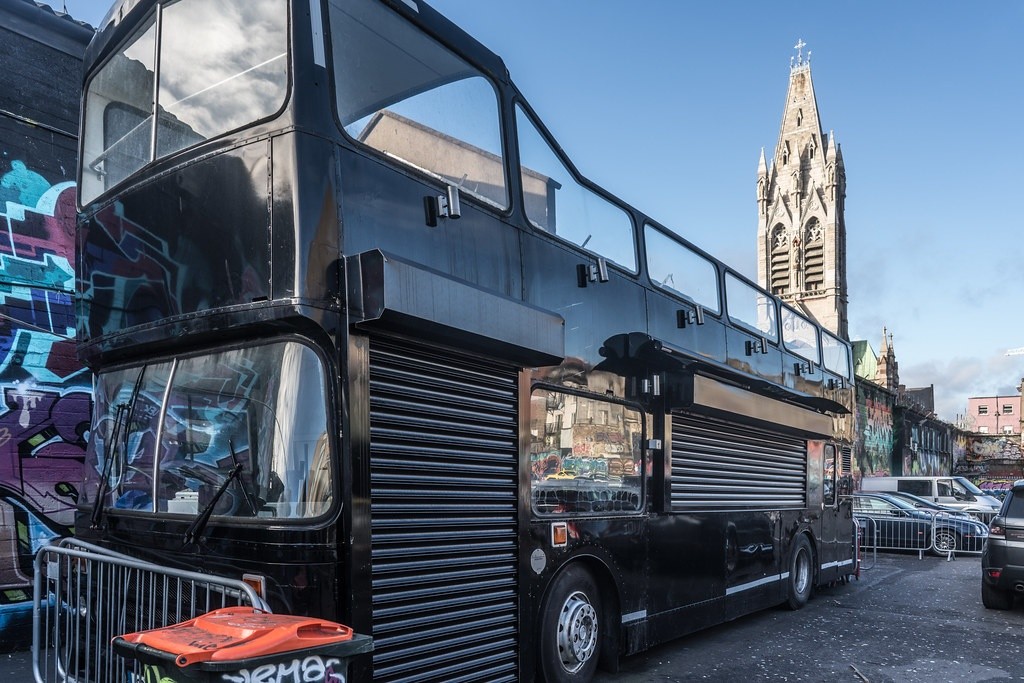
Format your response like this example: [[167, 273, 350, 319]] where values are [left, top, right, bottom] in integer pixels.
[[113, 605, 375, 682]]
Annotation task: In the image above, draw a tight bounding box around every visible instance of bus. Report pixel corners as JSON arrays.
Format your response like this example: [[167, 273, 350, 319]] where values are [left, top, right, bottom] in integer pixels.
[[62, 0, 862, 683]]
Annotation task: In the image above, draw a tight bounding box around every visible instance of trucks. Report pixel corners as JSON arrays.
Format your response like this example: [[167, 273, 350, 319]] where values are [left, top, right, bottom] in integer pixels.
[[861, 476, 1005, 527]]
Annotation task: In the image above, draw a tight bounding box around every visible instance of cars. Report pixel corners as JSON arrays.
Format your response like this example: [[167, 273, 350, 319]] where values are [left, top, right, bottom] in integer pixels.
[[851, 490, 989, 558]]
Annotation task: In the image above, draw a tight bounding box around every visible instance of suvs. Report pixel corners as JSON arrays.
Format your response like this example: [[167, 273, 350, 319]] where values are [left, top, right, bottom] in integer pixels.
[[980, 480, 1024, 611]]
